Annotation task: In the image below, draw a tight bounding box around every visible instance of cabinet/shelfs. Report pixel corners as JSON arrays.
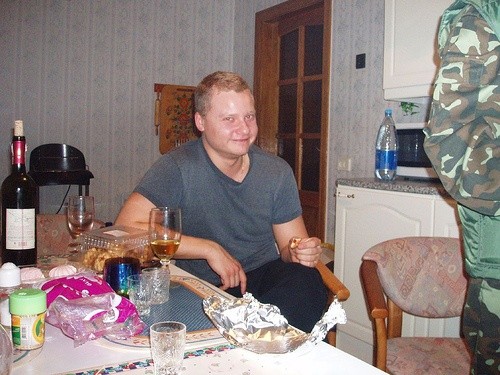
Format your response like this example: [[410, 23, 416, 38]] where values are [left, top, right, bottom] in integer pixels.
[[383, 0, 451, 104], [334, 186, 462, 337]]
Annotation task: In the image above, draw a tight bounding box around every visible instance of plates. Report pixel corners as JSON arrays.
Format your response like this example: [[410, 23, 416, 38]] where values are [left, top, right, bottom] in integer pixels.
[[15, 263, 86, 289]]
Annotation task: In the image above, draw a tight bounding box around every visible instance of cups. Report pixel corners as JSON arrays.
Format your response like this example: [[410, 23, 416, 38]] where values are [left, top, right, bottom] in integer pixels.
[[149, 321, 187, 375], [127, 273, 154, 316], [142, 267, 171, 304]]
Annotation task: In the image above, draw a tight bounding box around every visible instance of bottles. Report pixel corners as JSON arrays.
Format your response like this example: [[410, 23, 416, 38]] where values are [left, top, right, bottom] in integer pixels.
[[375, 109, 398, 183], [0, 325, 13, 375], [0, 262, 47, 351], [0, 120, 38, 268]]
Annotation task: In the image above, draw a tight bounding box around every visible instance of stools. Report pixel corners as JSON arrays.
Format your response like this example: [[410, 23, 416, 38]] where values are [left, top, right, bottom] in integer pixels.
[[27, 144, 94, 215]]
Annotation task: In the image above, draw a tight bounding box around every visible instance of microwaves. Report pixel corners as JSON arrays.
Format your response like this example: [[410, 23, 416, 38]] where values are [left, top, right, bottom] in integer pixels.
[[393, 123, 440, 183]]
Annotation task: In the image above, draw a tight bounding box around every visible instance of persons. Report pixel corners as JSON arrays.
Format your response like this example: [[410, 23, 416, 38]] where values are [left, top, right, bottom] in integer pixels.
[[423, 0, 500, 375], [113, 71, 327, 333]]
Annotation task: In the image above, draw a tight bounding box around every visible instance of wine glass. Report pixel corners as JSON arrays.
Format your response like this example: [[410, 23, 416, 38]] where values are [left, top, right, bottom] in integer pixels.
[[68, 195, 95, 253], [148, 206, 182, 292]]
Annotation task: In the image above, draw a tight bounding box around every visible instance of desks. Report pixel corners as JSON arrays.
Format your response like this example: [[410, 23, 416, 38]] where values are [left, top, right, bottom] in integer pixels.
[[0, 254, 390, 374]]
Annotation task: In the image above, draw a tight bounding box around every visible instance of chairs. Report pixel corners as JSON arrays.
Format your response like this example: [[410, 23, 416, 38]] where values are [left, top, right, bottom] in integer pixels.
[[359, 236, 473, 375], [36, 213, 105, 258]]
